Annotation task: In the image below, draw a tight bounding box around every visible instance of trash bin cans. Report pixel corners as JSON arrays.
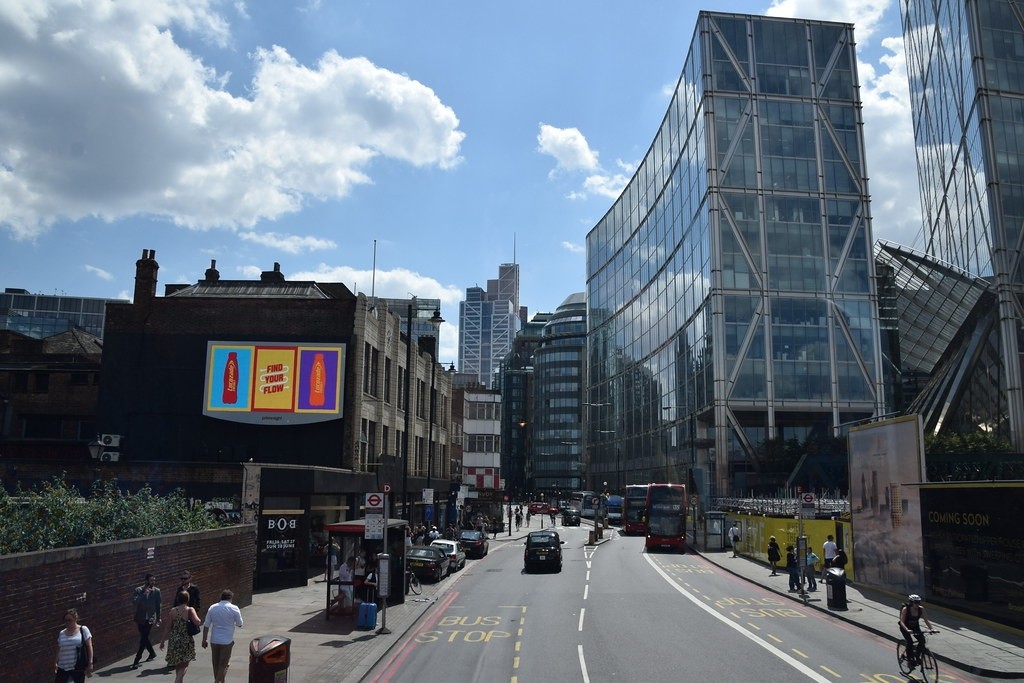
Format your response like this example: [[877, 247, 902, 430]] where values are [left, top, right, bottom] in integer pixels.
[[825, 567, 849, 611], [405, 571, 410, 594], [248, 635, 292, 683]]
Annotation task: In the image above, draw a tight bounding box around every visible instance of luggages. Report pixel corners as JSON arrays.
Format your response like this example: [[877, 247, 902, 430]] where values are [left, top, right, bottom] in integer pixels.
[[357, 586, 377, 629]]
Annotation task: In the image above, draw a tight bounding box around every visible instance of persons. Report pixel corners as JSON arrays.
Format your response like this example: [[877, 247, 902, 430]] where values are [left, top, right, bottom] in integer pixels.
[[202, 590, 243, 683], [173, 570, 202, 616], [54, 608, 94, 683], [787, 535, 847, 593], [322, 537, 380, 614], [897, 594, 938, 670], [550, 512, 556, 526], [767, 536, 782, 575], [131, 573, 162, 670], [406, 512, 498, 552], [160, 591, 200, 683], [506, 504, 531, 531], [728, 523, 741, 557]]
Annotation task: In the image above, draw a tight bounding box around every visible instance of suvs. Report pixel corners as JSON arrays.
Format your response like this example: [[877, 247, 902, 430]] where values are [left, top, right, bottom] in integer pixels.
[[529, 503, 558, 515]]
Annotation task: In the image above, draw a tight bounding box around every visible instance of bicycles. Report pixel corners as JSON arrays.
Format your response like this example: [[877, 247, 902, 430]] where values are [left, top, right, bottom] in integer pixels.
[[896, 630, 939, 683], [406, 563, 422, 595]]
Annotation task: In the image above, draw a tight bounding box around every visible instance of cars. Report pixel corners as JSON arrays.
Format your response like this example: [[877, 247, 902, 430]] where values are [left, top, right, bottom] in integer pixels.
[[561, 509, 581, 527], [456, 529, 490, 560], [524, 531, 565, 573], [407, 546, 453, 582], [432, 539, 466, 571]]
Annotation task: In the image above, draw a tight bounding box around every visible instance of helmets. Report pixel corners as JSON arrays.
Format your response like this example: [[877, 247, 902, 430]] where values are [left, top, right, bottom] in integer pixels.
[[909, 594, 921, 601]]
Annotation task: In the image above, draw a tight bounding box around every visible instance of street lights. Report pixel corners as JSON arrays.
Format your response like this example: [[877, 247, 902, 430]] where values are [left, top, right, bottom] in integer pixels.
[[561, 441, 577, 498], [508, 421, 525, 535], [663, 406, 697, 544], [583, 403, 613, 493]]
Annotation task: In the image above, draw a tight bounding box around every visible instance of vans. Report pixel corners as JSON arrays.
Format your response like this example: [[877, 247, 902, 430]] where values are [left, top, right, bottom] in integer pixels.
[[558, 501, 570, 511]]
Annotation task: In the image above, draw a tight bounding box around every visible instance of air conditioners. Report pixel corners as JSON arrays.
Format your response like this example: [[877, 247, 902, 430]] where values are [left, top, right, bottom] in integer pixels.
[[102, 434, 121, 447], [100, 452, 119, 462]]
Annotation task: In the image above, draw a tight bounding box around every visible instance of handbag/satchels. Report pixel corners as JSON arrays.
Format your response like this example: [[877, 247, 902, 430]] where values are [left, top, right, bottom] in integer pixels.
[[75, 624, 96, 670], [732, 535, 740, 542], [187, 607, 201, 635]]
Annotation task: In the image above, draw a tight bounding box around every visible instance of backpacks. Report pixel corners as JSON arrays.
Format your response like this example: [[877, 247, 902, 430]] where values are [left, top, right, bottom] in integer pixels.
[[900, 602, 911, 620]]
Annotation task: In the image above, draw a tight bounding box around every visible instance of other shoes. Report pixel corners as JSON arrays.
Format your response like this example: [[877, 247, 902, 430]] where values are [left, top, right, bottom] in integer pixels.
[[131, 664, 138, 670], [146, 652, 158, 661]]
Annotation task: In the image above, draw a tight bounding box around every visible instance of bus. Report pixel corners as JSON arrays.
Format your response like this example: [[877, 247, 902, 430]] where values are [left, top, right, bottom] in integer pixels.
[[570, 491, 598, 518], [620, 484, 649, 535], [642, 483, 692, 552]]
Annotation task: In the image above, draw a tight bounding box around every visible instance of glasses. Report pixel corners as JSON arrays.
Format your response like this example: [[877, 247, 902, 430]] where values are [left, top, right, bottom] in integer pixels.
[[181, 576, 191, 580]]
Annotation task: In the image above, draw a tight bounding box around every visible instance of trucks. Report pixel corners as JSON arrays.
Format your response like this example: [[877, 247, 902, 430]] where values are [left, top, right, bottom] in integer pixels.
[[598, 495, 624, 524]]
